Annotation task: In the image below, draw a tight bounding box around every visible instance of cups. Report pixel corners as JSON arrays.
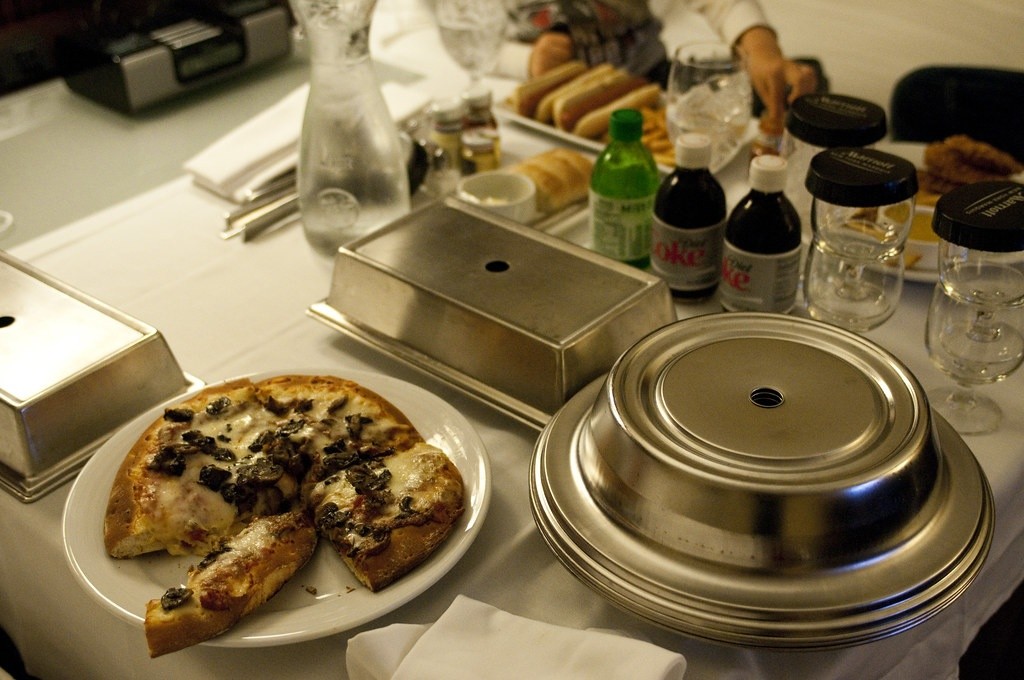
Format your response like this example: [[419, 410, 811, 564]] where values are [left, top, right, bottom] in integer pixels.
[[666, 42, 752, 170], [456, 170, 536, 226]]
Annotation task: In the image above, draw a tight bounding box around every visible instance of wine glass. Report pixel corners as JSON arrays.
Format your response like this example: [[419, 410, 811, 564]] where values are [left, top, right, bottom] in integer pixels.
[[924, 180, 1023, 434], [430, 1, 512, 92], [779, 91, 912, 332]]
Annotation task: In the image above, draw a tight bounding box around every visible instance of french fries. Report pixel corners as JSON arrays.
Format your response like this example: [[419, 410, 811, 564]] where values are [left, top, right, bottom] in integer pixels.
[[635, 107, 679, 168]]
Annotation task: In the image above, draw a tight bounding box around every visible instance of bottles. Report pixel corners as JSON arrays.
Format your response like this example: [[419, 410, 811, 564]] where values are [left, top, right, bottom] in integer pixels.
[[649, 133, 728, 301], [588, 109, 659, 273], [427, 88, 504, 183], [720, 153, 799, 317], [287, 1, 411, 248]]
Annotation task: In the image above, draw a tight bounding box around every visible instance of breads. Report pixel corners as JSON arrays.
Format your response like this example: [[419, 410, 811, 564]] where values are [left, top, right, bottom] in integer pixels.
[[509, 60, 662, 140], [508, 146, 595, 211]]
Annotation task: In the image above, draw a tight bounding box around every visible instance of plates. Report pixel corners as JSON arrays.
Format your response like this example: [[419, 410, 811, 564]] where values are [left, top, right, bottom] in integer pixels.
[[783, 144, 1022, 283], [64, 368, 489, 647], [528, 405, 997, 652], [491, 94, 759, 172]]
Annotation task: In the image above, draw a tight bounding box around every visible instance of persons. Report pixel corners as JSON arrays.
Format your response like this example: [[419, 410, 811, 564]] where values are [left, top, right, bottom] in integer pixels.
[[430, 1, 830, 120]]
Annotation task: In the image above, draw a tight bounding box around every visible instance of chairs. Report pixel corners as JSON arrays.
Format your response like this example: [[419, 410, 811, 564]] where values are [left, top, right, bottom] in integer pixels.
[[890, 62, 1024, 165]]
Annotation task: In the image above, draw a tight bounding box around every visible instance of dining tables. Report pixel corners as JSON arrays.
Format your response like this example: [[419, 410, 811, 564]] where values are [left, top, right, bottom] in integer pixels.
[[0, 68, 1024, 680]]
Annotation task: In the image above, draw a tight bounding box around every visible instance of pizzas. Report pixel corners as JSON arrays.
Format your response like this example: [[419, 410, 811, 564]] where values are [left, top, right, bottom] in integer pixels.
[[103, 374, 465, 658]]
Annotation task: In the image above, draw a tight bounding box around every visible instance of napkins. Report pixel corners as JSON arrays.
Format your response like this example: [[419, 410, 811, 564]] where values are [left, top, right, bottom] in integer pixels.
[[184, 76, 312, 202]]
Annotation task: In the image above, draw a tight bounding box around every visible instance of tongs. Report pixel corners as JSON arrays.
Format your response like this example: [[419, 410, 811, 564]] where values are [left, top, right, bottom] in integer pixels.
[[221, 180, 299, 241]]
